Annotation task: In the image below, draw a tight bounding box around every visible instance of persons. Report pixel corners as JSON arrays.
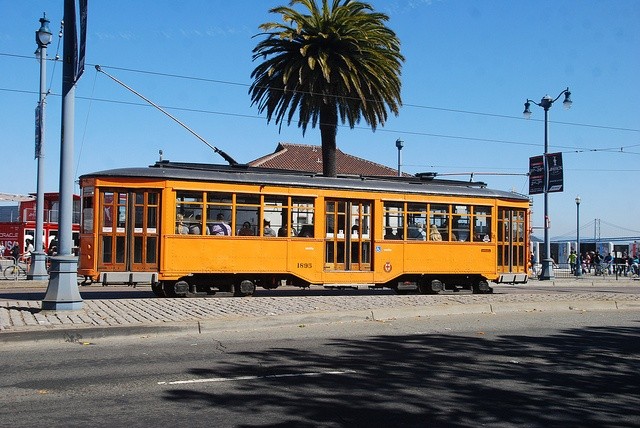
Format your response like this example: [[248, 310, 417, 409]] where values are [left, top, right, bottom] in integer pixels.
[[405, 223, 423, 240], [416, 222, 426, 240], [237, 222, 255, 235], [264, 219, 276, 237], [9, 242, 19, 274], [528, 251, 539, 271], [48, 237, 60, 256], [211, 213, 232, 236], [550, 253, 559, 269], [384, 227, 396, 239], [391, 227, 404, 239], [189, 215, 210, 235], [444, 224, 457, 241], [22, 239, 35, 258], [429, 225, 443, 241], [278, 220, 298, 237], [565, 250, 640, 278], [176, 213, 190, 234]]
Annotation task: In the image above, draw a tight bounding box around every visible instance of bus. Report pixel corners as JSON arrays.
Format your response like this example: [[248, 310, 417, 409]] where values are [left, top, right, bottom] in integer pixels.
[[78, 166, 532, 294]]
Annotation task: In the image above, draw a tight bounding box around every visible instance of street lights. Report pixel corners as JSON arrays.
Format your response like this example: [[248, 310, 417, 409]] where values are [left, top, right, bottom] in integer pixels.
[[522, 86, 573, 280], [575, 194, 582, 275], [27, 10, 55, 279], [395, 136, 405, 176]]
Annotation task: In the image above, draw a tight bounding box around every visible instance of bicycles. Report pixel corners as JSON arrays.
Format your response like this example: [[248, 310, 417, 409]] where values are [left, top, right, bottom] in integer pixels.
[[3, 252, 31, 279]]
